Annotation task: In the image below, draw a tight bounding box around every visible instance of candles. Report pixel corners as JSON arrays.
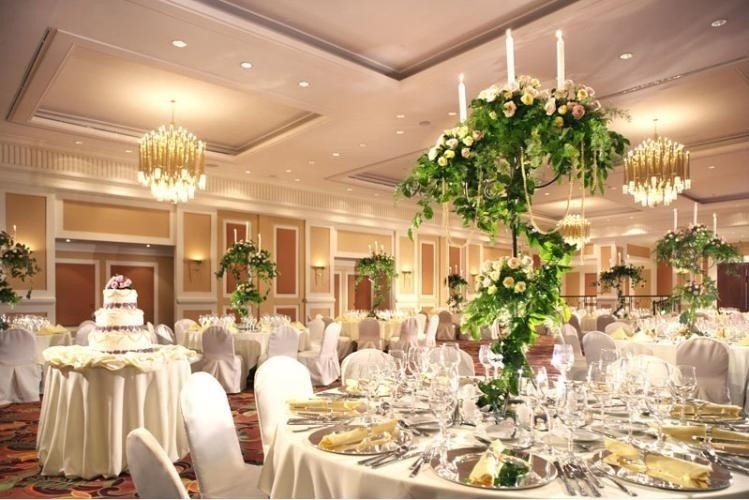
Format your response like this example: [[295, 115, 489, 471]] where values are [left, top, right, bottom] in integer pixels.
[[367, 241, 386, 255], [693, 201, 698, 225], [257, 234, 262, 255], [555, 30, 566, 91], [233, 227, 238, 243], [505, 28, 515, 84], [457, 74, 467, 123], [673, 208, 678, 232], [713, 213, 717, 235]]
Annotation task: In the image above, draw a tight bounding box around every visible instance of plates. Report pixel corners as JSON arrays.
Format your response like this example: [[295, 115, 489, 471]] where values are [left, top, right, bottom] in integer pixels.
[[286, 387, 749, 491]]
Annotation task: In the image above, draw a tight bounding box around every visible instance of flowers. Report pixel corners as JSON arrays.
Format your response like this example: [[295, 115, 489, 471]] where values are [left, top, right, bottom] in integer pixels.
[[215, 240, 282, 322], [654, 224, 743, 339], [390, 76, 631, 408], [353, 253, 400, 319], [0, 230, 43, 330], [591, 262, 646, 319]]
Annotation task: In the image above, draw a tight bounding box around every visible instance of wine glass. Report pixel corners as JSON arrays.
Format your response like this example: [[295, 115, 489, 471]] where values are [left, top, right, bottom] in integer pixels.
[[645, 307, 742, 339], [357, 341, 697, 464], [198, 311, 289, 332], [0, 314, 49, 332]]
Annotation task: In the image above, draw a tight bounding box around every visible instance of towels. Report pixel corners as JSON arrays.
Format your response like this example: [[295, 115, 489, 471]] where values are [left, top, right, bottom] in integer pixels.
[[39, 345, 198, 371], [184, 318, 307, 334]]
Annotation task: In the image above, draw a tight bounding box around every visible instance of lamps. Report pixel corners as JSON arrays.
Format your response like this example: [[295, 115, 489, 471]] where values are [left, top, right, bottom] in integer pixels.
[[138, 101, 206, 204], [471, 270, 480, 279], [622, 117, 693, 207], [555, 214, 592, 251], [312, 264, 326, 276], [403, 267, 413, 277], [188, 256, 208, 271]]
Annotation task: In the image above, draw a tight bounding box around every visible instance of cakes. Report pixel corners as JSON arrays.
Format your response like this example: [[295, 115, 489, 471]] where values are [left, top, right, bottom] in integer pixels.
[[87, 274, 156, 355]]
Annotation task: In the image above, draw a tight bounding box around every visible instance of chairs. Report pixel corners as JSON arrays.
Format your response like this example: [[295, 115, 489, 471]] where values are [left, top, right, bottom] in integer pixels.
[[253, 356, 314, 457], [180, 371, 270, 499], [126, 428, 189, 498]]
